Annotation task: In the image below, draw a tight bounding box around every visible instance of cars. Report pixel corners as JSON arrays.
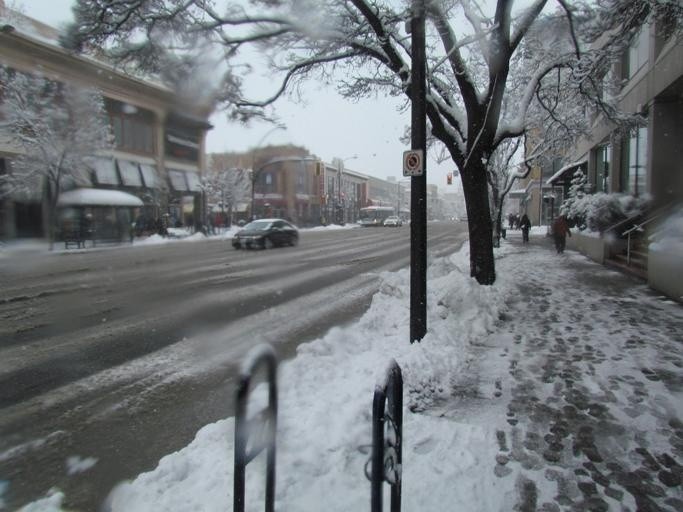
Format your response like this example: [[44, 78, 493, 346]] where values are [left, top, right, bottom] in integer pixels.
[[231, 218, 303, 250], [382, 215, 403, 227]]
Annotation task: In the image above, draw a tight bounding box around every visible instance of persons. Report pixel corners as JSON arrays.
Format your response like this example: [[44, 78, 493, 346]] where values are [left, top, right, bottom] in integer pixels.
[[552, 216, 571, 255], [509, 212, 532, 243]]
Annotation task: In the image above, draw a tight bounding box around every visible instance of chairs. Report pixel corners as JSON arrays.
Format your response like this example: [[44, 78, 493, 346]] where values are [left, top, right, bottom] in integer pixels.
[[61, 220, 96, 249]]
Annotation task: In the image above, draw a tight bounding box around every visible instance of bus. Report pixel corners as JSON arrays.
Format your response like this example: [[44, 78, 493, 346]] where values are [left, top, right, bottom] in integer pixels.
[[359, 206, 397, 228]]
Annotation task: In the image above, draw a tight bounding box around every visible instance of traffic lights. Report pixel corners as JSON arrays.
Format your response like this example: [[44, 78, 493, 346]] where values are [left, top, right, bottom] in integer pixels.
[[446, 172, 452, 185]]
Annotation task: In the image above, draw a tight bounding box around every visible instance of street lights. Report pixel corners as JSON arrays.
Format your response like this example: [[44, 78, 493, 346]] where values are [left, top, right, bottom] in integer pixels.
[[248, 123, 288, 227], [336, 154, 362, 228]]
